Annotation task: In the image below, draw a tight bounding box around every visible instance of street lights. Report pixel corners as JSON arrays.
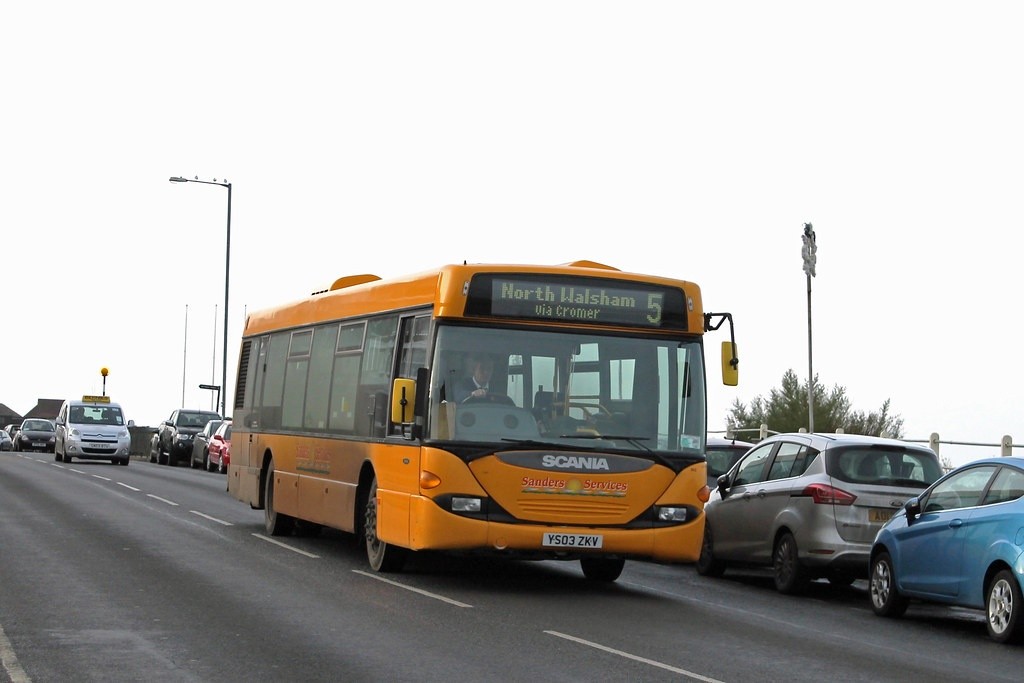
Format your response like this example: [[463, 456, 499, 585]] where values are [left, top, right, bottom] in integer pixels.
[[170, 175, 233, 419]]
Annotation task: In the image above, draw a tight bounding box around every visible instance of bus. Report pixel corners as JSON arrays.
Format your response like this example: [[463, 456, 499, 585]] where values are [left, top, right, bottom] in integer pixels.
[[226, 259, 741, 585]]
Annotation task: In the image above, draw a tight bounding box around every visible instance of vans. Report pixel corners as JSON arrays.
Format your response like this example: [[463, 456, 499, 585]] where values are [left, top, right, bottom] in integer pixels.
[[54, 395, 134, 466]]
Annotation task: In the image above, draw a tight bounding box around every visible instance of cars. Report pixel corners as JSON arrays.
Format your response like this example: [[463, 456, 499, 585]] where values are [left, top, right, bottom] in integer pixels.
[[190, 417, 224, 470], [0, 419, 56, 453], [206, 421, 233, 473], [149, 420, 167, 463], [867, 456, 1024, 643]]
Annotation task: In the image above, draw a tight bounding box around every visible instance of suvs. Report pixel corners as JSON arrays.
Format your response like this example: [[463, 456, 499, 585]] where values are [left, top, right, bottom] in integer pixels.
[[705, 436, 787, 493], [692, 432, 965, 595], [156, 406, 222, 466]]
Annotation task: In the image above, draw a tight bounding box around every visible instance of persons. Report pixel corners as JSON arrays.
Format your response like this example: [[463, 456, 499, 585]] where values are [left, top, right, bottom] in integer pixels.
[[452, 354, 504, 404]]
